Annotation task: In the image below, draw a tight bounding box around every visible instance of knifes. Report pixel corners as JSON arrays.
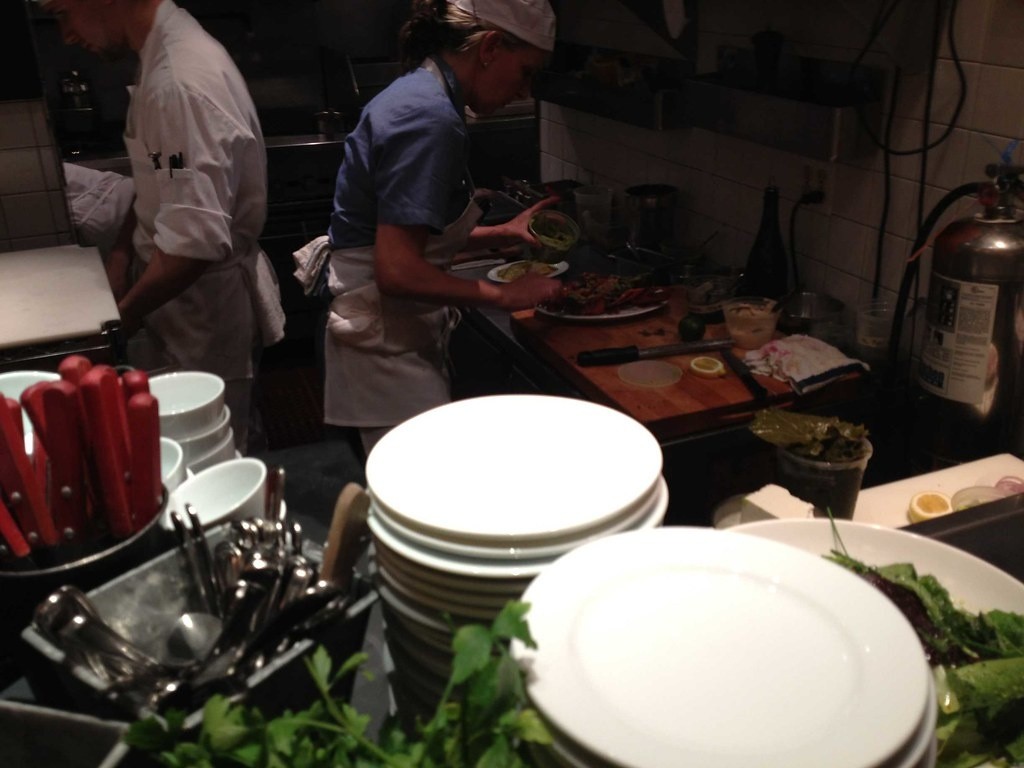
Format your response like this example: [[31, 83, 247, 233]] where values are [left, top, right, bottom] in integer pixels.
[[578, 339, 736, 365]]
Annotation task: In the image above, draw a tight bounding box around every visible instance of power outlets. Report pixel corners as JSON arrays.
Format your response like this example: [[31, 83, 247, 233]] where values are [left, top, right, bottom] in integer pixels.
[[800, 163, 835, 215]]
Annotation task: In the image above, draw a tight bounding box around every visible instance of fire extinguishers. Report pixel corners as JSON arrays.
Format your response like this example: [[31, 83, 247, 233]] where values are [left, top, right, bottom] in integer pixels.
[[882, 163, 1024, 467]]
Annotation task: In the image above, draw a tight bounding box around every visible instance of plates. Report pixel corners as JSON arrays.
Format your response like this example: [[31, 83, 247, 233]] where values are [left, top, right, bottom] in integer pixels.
[[358, 394, 1024, 768], [537, 300, 669, 322], [488, 259, 569, 283]]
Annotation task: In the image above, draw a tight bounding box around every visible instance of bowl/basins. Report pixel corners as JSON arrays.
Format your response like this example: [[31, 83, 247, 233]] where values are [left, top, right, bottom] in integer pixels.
[[0, 369, 286, 543], [528, 209, 580, 264], [725, 297, 782, 349], [783, 292, 841, 345]]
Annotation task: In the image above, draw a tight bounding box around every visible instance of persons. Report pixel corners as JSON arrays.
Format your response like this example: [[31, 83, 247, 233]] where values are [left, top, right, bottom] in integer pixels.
[[37, 0, 286, 460], [318, 1, 566, 474]]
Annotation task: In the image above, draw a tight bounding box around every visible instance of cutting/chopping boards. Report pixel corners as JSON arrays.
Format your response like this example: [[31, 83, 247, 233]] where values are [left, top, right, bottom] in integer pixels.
[[510, 285, 798, 439]]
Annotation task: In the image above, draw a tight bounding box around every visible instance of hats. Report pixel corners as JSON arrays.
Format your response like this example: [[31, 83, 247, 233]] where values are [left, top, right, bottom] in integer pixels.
[[446, 0, 557, 52], [31, 0, 51, 7]]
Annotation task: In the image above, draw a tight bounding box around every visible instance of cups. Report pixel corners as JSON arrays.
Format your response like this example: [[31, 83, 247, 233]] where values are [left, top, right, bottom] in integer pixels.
[[783, 438, 873, 520], [624, 184, 676, 248], [858, 297, 920, 387], [575, 185, 613, 241]]
